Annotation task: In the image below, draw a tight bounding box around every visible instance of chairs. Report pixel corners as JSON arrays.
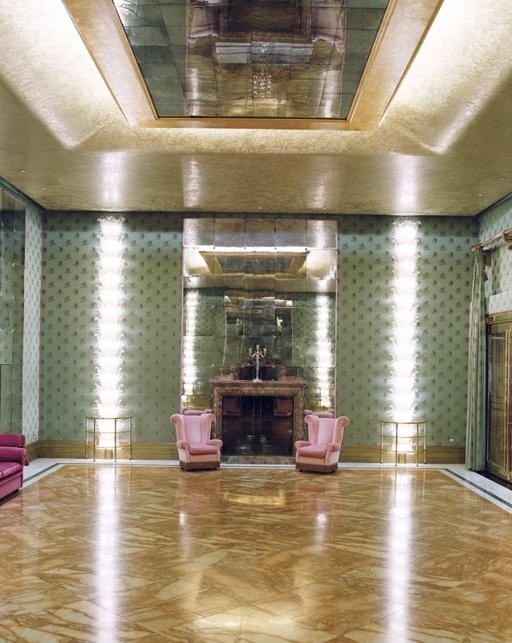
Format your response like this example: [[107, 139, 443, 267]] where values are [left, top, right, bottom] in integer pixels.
[[293, 413, 350, 473], [169, 412, 225, 472]]
